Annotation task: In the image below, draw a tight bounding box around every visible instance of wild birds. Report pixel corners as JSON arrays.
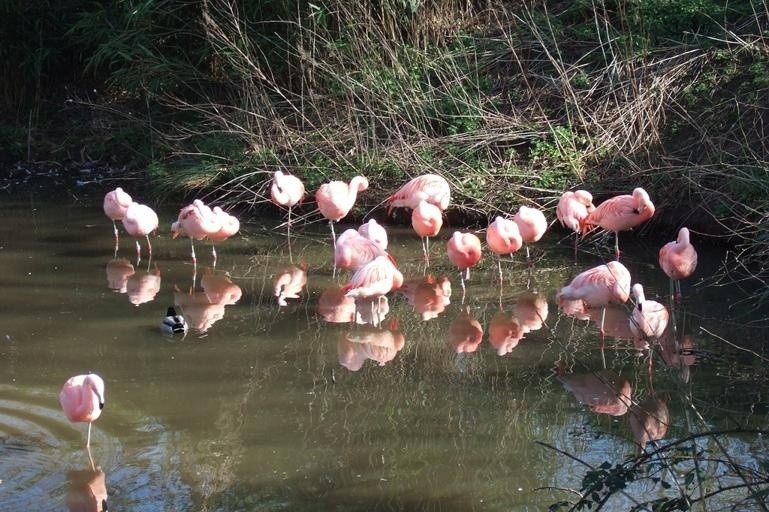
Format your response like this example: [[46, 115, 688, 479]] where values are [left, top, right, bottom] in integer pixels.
[[316, 172, 368, 221], [517, 205, 546, 244], [270, 170, 303, 224], [106, 237, 162, 308], [585, 187, 656, 256], [487, 215, 522, 253], [658, 227, 698, 294], [412, 200, 443, 250], [63, 447, 108, 512], [102, 188, 159, 262], [170, 199, 241, 262], [448, 231, 481, 272], [273, 248, 548, 373], [630, 282, 669, 365], [386, 173, 451, 211], [335, 229, 381, 266], [557, 346, 632, 417], [161, 306, 188, 333], [556, 190, 596, 260], [628, 365, 669, 453], [58, 374, 105, 447], [360, 219, 388, 250], [172, 260, 242, 338], [557, 260, 631, 336]]
